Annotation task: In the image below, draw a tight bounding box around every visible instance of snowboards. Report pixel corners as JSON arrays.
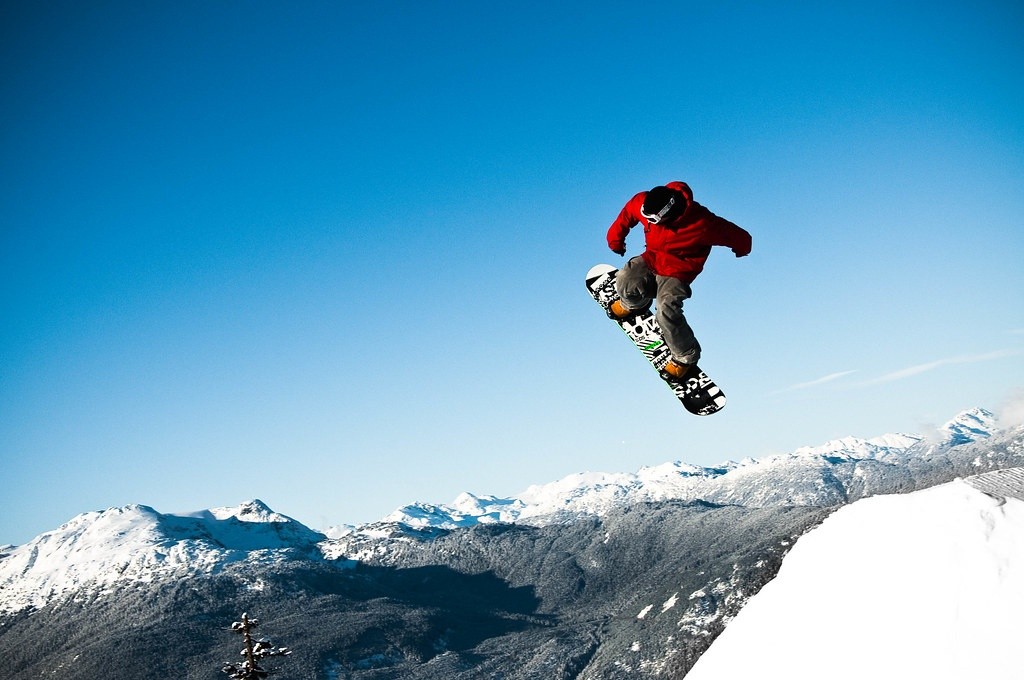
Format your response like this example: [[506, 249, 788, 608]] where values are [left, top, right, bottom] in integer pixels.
[[584, 264, 728, 416]]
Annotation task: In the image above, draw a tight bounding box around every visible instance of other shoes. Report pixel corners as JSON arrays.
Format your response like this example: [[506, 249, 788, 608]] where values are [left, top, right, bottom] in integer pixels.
[[661, 358, 697, 380], [607, 299, 653, 320]]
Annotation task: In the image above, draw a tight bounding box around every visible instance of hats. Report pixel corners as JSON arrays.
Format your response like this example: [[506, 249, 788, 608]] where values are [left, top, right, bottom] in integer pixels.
[[644, 186, 678, 218]]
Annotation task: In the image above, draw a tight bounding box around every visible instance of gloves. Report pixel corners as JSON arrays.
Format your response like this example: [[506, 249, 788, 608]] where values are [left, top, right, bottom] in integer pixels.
[[615, 243, 626, 257]]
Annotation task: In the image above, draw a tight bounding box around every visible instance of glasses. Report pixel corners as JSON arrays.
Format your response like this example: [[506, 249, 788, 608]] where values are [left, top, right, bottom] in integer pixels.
[[640, 204, 661, 224]]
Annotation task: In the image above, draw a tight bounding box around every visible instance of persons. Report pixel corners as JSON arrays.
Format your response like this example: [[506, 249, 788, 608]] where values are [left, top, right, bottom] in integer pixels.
[[607, 182, 751, 381]]
